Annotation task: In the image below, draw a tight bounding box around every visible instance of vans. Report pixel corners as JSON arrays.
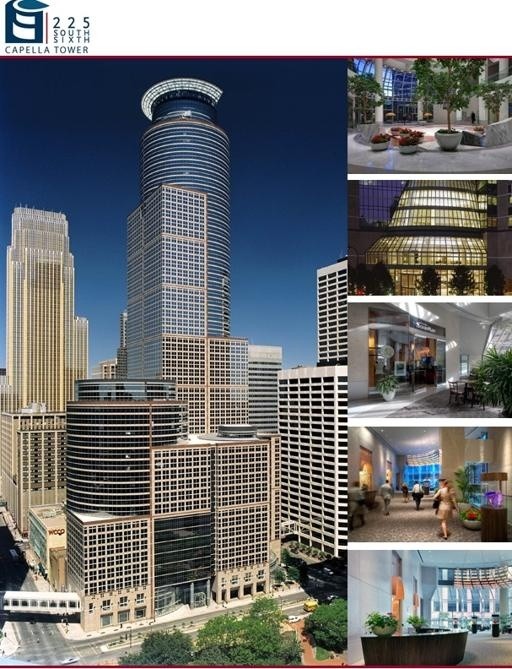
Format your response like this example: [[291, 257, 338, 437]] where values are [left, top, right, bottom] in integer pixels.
[[304, 601, 318, 612]]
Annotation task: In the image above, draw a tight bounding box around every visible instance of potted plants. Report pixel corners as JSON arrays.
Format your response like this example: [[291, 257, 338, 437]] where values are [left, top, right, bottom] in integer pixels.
[[347, 57, 495, 155], [407, 616, 427, 628], [363, 612, 401, 637], [452, 464, 474, 516], [375, 373, 399, 402]]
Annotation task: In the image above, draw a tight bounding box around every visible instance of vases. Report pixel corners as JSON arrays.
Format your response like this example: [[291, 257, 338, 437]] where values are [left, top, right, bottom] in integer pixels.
[[463, 519, 483, 531]]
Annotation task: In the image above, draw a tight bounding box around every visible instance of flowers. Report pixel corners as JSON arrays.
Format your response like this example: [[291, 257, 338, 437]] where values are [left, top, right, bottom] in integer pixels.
[[457, 505, 483, 521]]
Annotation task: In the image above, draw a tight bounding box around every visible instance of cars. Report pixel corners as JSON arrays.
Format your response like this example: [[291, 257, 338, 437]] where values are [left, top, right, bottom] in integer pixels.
[[291, 549, 298, 554], [287, 615, 301, 623], [323, 567, 334, 575], [326, 594, 338, 601], [60, 656, 80, 664]]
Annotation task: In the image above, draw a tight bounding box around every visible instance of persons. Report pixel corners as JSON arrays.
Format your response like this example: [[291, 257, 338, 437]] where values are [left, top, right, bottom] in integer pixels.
[[401, 483, 409, 503], [379, 480, 391, 516], [349, 481, 365, 532], [412, 480, 423, 510], [432, 478, 458, 538]]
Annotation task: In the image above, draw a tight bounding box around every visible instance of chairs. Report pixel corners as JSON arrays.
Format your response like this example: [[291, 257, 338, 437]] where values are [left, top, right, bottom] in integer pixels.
[[448, 374, 502, 412]]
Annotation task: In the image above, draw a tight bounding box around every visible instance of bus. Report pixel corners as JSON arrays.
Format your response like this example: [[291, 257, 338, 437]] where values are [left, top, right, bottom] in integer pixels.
[[9, 549, 19, 562]]
